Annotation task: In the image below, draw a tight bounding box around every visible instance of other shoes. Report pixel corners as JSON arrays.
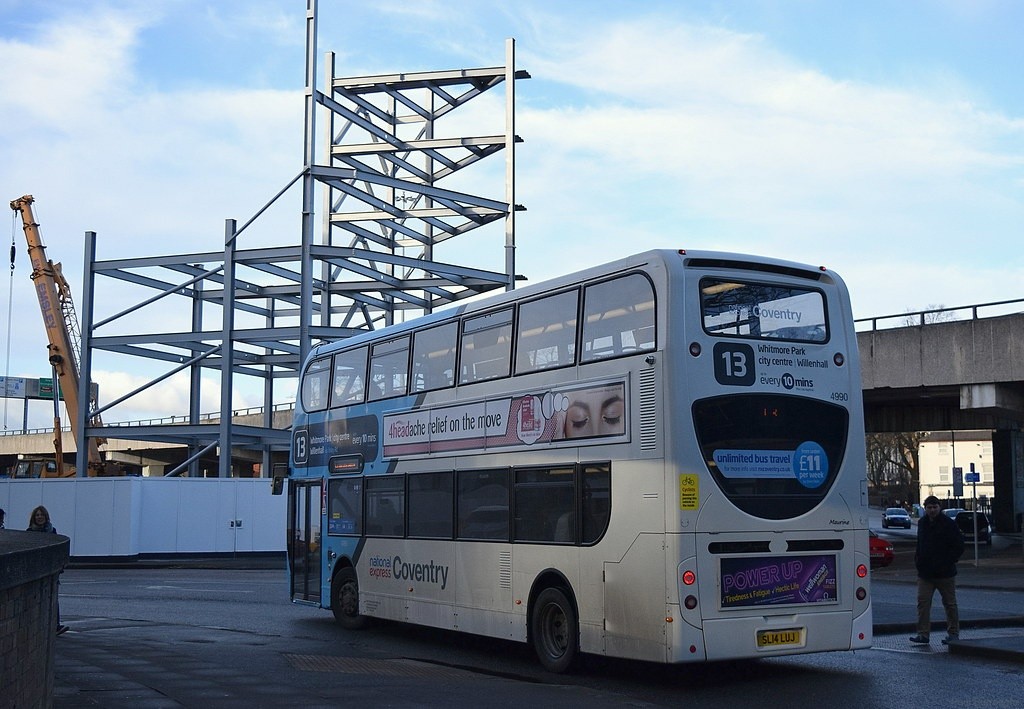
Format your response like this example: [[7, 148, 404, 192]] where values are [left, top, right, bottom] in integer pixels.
[[909, 634, 929, 644], [941, 636, 959, 645], [56, 624, 70, 634]]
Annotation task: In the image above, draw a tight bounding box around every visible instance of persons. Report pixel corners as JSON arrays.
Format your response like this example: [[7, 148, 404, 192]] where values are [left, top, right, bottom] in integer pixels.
[[909, 496, 965, 644], [375, 499, 403, 536], [556, 383, 624, 438], [0, 508, 6, 529], [26, 506, 69, 635]]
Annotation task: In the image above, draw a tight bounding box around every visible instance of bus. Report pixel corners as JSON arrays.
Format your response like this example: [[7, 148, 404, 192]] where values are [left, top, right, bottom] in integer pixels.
[[272, 248, 872, 679]]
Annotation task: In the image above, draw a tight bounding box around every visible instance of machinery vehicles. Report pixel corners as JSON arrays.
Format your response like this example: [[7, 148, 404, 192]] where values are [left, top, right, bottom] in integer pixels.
[[8, 194, 127, 479]]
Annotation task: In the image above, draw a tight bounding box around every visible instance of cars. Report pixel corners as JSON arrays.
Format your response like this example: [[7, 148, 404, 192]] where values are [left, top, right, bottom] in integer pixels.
[[954, 511, 992, 545], [869, 528, 892, 568], [942, 509, 968, 521], [883, 507, 912, 528]]
